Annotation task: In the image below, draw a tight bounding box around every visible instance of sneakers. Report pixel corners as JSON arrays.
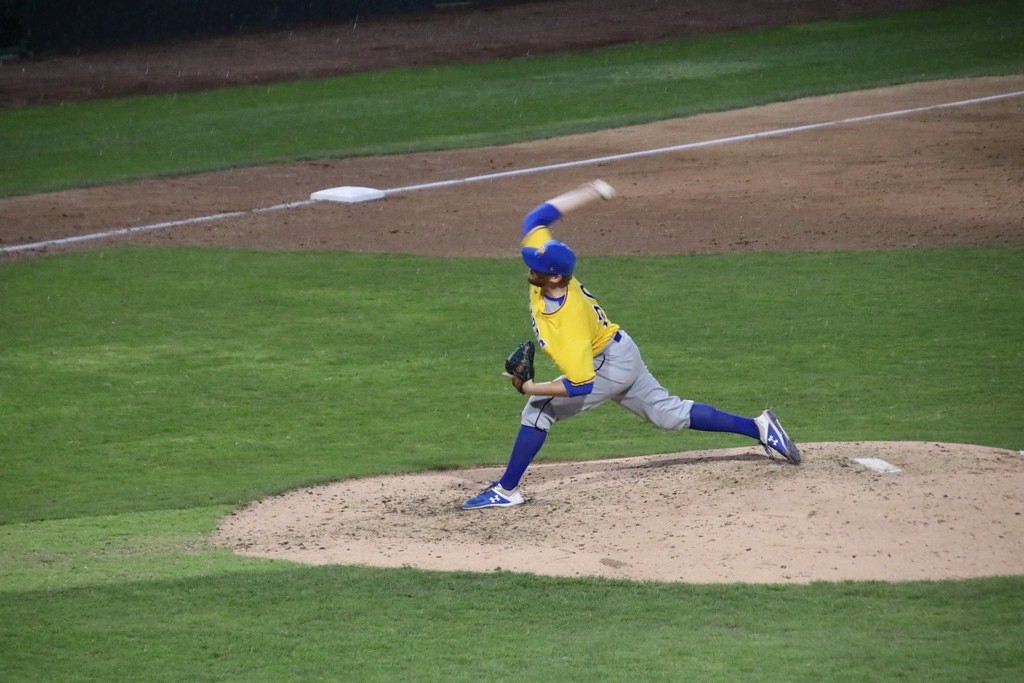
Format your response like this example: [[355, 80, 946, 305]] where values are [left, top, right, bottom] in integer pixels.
[[753, 409, 800, 464], [461, 480, 524, 510]]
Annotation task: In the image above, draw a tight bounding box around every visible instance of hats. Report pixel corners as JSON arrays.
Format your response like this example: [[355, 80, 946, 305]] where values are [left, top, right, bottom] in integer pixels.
[[521, 240, 576, 276]]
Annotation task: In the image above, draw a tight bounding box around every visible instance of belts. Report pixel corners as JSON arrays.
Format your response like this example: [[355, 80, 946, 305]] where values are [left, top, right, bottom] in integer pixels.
[[613, 331, 621, 342]]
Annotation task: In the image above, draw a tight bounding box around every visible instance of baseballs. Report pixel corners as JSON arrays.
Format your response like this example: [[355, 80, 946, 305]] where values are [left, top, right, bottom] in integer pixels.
[[603, 185, 615, 199]]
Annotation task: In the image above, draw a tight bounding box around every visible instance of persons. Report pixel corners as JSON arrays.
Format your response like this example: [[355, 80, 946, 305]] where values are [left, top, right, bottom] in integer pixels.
[[461, 182, 802, 509]]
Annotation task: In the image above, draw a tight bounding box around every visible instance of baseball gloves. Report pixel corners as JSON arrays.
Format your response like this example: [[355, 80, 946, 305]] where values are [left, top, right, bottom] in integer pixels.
[[504, 340, 535, 395]]
